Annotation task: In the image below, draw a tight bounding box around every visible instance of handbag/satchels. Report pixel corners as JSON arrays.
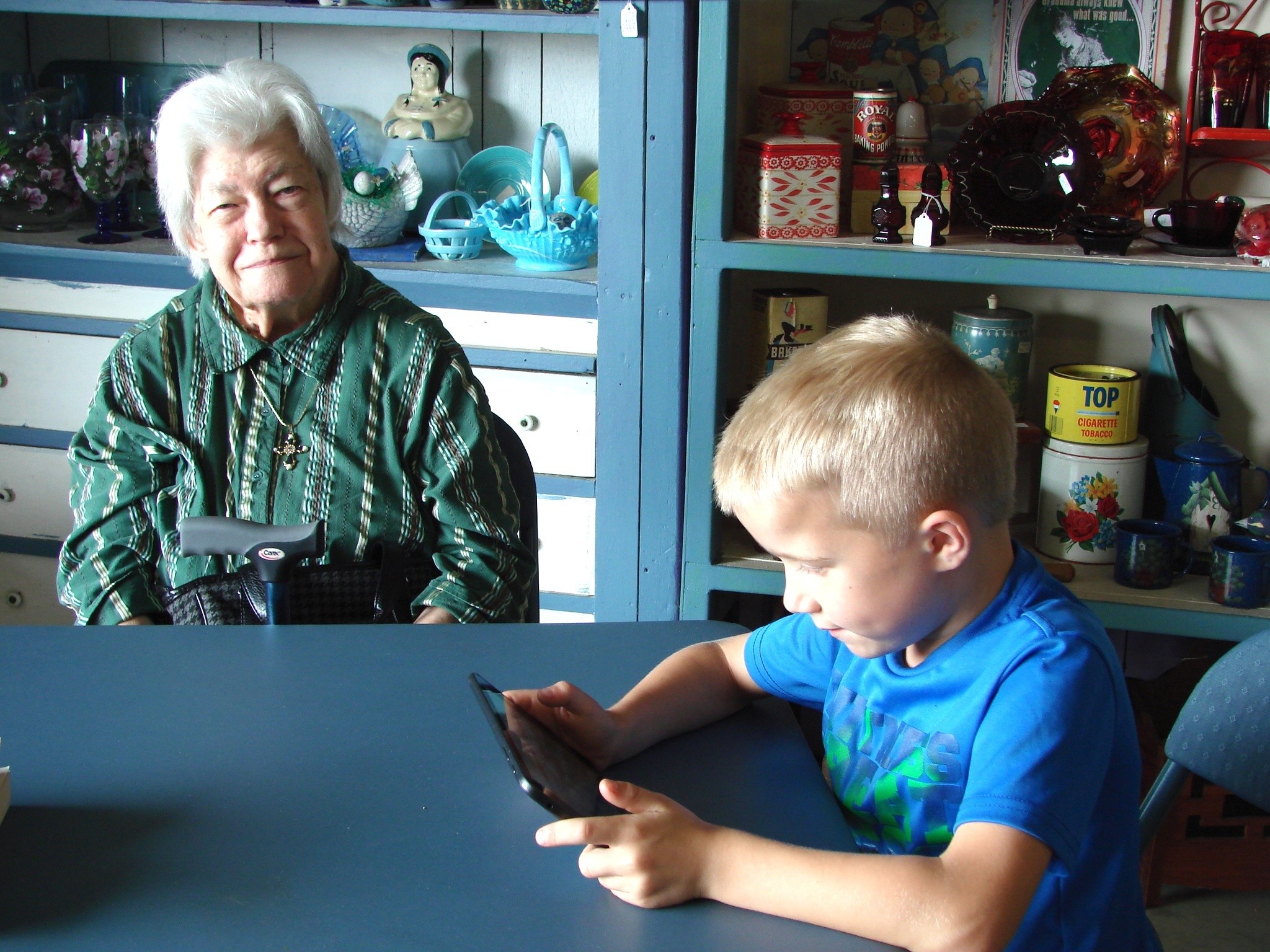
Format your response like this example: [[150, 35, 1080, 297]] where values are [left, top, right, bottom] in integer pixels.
[[159, 541, 437, 624]]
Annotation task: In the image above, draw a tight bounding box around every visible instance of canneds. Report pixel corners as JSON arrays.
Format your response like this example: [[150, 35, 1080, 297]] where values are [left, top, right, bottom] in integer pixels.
[[852, 89, 897, 164]]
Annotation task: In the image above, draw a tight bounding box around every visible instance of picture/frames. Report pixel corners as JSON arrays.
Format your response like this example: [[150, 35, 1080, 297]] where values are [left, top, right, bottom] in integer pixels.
[[986, 1, 1169, 119]]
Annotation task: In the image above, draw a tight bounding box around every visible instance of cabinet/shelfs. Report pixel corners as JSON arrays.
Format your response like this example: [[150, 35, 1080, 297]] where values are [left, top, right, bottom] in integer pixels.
[[681, 1, 1270, 641], [1, 0, 682, 620]]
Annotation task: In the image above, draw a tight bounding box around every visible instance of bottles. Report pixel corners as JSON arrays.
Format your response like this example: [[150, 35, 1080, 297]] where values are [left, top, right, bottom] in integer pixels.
[[739, 112, 844, 240], [0, 90, 85, 232], [759, 60, 853, 228]]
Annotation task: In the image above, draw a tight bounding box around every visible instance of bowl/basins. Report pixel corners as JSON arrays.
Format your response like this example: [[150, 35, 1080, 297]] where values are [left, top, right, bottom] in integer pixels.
[[1068, 212, 1144, 256]]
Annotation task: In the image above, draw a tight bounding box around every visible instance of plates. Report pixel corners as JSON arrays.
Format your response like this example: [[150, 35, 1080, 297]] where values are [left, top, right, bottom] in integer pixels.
[[950, 99, 1101, 241], [1036, 63, 1180, 217], [1143, 232, 1237, 257]]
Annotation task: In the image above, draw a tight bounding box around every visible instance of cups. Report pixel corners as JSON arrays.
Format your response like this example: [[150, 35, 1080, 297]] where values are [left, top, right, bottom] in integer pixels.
[[1111, 515, 1188, 591], [1207, 533, 1269, 608], [1152, 193, 1245, 249], [1029, 430, 1149, 565], [0, 72, 34, 103], [1198, 30, 1258, 127], [318, 0, 597, 16], [1258, 32, 1270, 128], [952, 293, 1034, 421]]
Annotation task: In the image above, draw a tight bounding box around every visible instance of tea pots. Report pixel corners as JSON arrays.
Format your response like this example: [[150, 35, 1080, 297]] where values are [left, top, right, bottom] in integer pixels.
[[1145, 431, 1270, 577]]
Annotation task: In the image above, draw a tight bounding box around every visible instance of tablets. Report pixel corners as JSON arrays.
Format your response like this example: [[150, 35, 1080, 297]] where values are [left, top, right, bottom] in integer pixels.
[[466, 673, 625, 849]]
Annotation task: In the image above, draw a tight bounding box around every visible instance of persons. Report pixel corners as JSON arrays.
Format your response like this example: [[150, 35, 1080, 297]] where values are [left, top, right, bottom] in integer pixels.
[[378, 43, 475, 230], [498, 313, 1161, 952], [57, 60, 535, 627]]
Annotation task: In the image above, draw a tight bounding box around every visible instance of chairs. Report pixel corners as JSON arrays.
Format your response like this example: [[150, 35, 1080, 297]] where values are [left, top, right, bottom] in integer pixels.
[[1133, 628, 1270, 856]]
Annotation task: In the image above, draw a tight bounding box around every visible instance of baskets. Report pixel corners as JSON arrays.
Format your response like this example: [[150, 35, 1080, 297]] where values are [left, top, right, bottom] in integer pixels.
[[471, 123, 598, 272], [418, 190, 487, 261]]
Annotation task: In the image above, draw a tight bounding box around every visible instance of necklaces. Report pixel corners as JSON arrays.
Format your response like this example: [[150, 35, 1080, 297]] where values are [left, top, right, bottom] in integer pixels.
[[249, 362, 320, 469]]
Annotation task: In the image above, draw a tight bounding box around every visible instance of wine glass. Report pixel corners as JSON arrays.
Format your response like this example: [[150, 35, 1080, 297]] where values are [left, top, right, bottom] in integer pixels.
[[69, 117, 132, 245], [108, 70, 157, 230], [46, 70, 97, 221]]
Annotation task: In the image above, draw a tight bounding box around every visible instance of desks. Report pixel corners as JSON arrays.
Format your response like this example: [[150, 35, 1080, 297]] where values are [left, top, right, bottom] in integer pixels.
[[0, 620, 906, 951]]
